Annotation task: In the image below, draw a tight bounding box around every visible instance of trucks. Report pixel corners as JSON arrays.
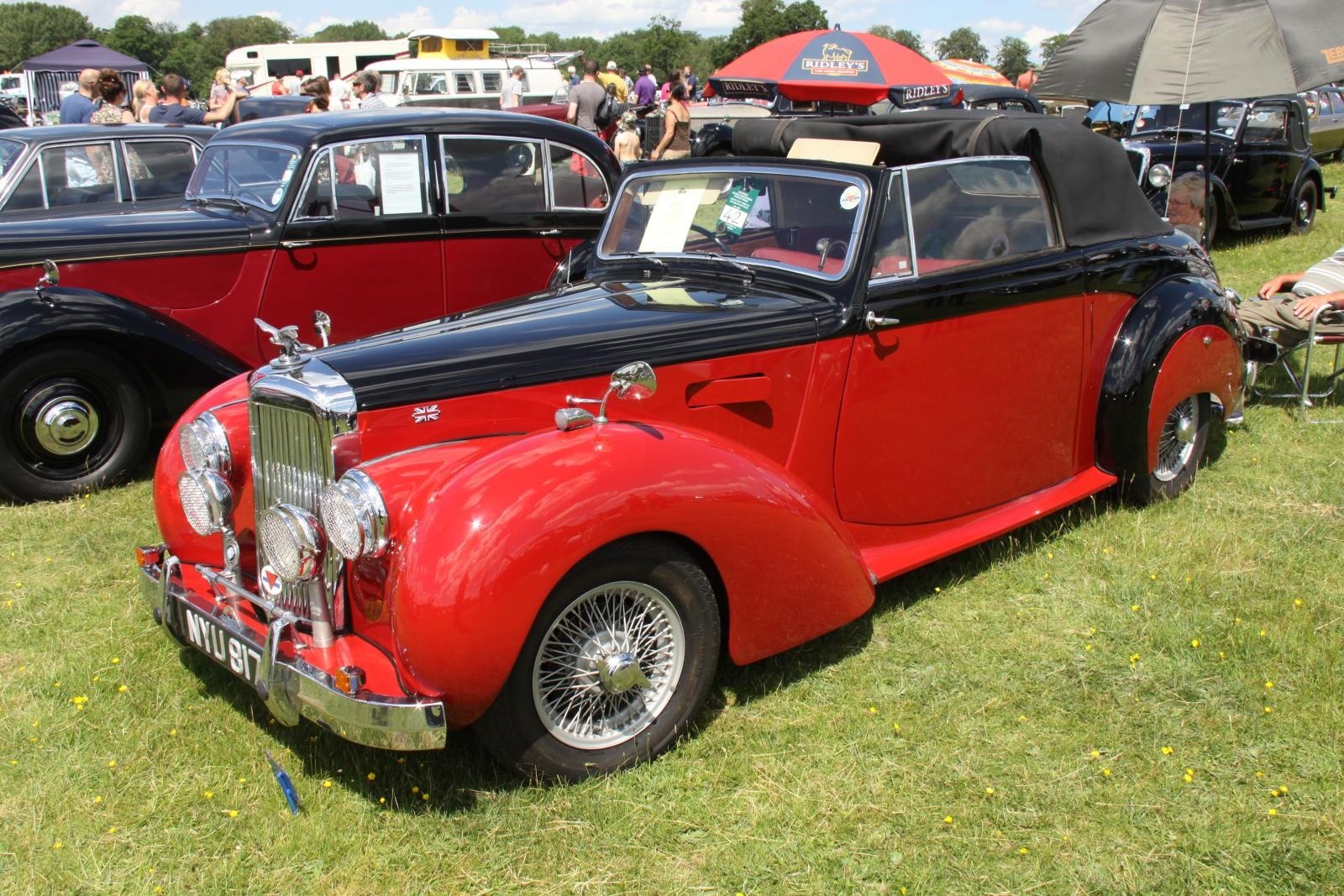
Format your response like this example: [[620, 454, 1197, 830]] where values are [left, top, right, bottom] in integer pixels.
[[351, 41, 583, 107], [221, 37, 409, 98]]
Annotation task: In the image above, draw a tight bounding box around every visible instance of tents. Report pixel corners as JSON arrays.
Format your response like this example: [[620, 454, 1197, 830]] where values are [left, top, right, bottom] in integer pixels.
[[9, 40, 151, 127]]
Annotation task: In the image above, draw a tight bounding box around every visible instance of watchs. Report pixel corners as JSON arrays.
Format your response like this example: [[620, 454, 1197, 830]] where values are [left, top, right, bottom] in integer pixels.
[[98, 172, 104, 175]]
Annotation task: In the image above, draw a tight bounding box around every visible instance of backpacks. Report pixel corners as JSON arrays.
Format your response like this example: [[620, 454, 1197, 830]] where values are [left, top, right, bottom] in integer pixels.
[[594, 83, 618, 130]]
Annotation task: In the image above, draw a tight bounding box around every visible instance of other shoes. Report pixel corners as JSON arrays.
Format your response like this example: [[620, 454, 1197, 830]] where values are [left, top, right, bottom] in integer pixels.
[[1225, 410, 1244, 429]]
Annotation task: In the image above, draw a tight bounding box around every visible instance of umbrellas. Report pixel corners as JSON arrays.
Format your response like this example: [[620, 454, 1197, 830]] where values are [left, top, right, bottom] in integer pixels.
[[928, 59, 1014, 90], [1030, 0, 1344, 106], [706, 28, 963, 114]]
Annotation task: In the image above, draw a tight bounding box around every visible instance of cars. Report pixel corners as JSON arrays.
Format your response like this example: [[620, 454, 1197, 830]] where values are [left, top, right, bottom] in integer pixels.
[[137, 83, 1253, 782], [0, 94, 622, 502], [1082, 81, 1344, 253]]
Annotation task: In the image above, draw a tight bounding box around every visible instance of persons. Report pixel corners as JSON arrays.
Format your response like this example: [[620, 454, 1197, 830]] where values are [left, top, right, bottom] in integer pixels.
[[60, 68, 107, 187], [1216, 247, 1344, 424], [568, 59, 608, 142], [499, 66, 523, 109], [289, 70, 353, 185], [90, 68, 155, 184], [614, 111, 643, 201], [652, 81, 692, 160], [571, 61, 697, 106], [1163, 172, 1213, 244], [272, 72, 289, 95], [208, 67, 253, 129], [149, 73, 249, 124], [349, 68, 396, 216], [131, 80, 160, 123]]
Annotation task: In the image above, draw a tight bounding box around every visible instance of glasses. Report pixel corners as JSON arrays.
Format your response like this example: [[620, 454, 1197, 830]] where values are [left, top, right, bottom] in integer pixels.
[[1165, 197, 1192, 211], [351, 81, 360, 87], [666, 90, 672, 93]]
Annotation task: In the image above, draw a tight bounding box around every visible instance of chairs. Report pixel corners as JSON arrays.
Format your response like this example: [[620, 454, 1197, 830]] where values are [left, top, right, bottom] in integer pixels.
[[1250, 283, 1344, 423], [878, 256, 981, 276], [50, 188, 115, 206], [750, 247, 877, 278]]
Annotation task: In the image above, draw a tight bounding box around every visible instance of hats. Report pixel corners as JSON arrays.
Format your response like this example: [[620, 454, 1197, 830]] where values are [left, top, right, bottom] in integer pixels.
[[605, 62, 618, 72], [235, 70, 252, 81], [637, 66, 647, 73], [616, 111, 638, 131], [296, 70, 303, 76]]
